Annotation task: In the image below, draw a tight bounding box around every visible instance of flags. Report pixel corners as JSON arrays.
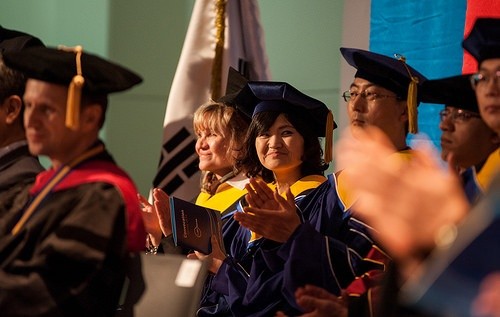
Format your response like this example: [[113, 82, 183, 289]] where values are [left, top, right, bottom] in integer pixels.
[[152, 0, 271, 204]]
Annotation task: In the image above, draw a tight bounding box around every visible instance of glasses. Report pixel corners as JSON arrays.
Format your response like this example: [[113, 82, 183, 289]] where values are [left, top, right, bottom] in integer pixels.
[[439, 109, 480, 123], [469, 67, 500, 89], [341, 89, 402, 103]]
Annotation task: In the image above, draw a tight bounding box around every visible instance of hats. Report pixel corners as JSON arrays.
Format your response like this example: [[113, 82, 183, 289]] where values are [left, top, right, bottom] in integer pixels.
[[1, 45, 144, 130], [219, 81, 337, 166], [339, 46, 429, 133], [461, 17, 500, 63], [425, 74, 479, 111]]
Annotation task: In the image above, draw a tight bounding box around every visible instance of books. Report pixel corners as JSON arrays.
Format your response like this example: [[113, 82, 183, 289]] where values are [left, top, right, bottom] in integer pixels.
[[169, 196, 227, 258]]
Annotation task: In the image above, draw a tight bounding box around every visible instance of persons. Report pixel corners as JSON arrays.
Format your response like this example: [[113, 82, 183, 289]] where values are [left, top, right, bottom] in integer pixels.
[[0, 25, 45, 238], [233, 46, 432, 317], [0, 47, 147, 317], [295, 15, 500, 316], [186, 80, 337, 317], [137, 66, 259, 258]]
